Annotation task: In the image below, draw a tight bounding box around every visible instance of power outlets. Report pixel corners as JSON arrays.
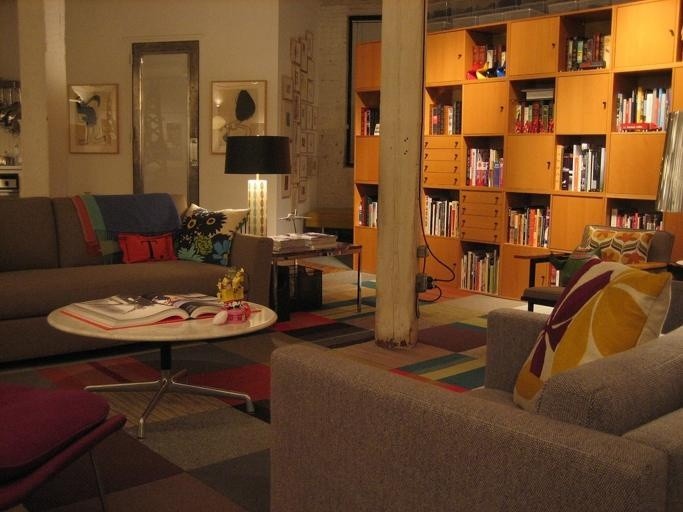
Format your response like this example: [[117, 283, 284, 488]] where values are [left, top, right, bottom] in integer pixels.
[[416, 271, 430, 293]]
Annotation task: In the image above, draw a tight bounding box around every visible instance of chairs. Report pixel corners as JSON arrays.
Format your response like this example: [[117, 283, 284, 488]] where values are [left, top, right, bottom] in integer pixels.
[[520, 224, 675, 312], [0, 381, 127, 512], [270, 308, 683, 512]]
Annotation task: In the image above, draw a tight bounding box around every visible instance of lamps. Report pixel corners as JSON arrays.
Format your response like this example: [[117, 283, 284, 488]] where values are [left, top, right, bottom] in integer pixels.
[[224, 136, 291, 237]]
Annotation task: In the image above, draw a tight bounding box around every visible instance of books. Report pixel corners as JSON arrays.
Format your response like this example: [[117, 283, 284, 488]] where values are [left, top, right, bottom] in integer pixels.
[[616, 84, 668, 133], [430, 102, 461, 135], [360, 107, 383, 138], [563, 36, 612, 70], [557, 140, 602, 192], [515, 91, 555, 134], [471, 42, 509, 78], [610, 209, 665, 235], [466, 148, 503, 189], [508, 203, 551, 251], [462, 250, 498, 295], [544, 262, 566, 289], [361, 193, 383, 228], [424, 193, 459, 239], [63, 290, 261, 332], [267, 232, 338, 253]]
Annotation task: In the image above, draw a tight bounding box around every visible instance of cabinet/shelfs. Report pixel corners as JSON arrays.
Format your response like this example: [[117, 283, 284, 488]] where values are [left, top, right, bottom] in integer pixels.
[[353, 1, 683, 301]]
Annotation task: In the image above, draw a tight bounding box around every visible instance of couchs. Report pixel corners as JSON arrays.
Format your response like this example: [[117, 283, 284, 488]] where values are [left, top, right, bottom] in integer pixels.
[[0, 193, 274, 364]]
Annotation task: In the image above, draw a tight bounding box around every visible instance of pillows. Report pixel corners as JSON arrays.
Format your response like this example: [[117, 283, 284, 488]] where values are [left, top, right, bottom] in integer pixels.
[[513, 253, 672, 415], [117, 231, 179, 264], [174, 203, 251, 265], [581, 226, 657, 264], [0, 385, 108, 477], [559, 246, 601, 288]]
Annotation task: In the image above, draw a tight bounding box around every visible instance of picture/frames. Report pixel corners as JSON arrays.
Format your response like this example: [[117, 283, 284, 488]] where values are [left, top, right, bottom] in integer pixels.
[[282, 30, 318, 214], [67, 84, 119, 154], [211, 80, 267, 154]]
